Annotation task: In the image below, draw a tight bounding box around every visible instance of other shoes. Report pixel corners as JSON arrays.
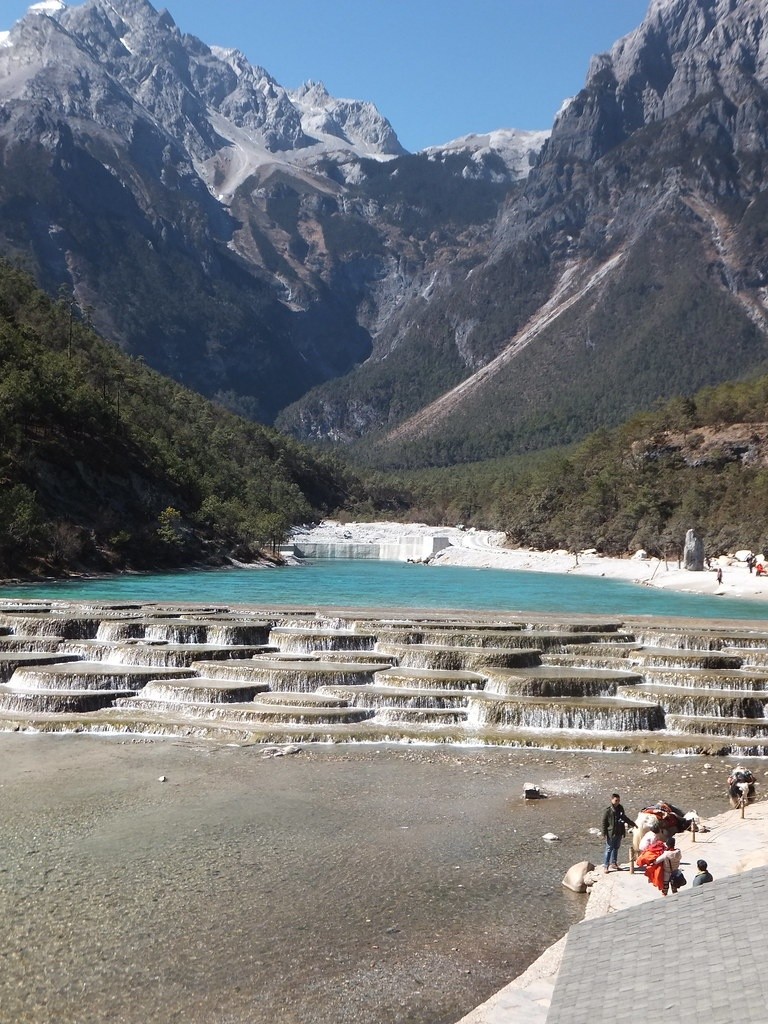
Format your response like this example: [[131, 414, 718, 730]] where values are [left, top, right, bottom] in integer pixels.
[[612, 864, 621, 869], [604, 867, 609, 874]]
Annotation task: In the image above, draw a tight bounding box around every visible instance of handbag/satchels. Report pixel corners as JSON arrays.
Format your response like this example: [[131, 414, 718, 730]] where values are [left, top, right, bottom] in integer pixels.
[[671, 871, 686, 888]]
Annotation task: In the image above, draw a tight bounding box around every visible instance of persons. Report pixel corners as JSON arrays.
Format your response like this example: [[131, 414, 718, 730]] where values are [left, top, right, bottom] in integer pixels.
[[650, 838, 682, 895], [693, 860, 713, 887], [717, 568, 724, 585], [602, 794, 638, 874]]
